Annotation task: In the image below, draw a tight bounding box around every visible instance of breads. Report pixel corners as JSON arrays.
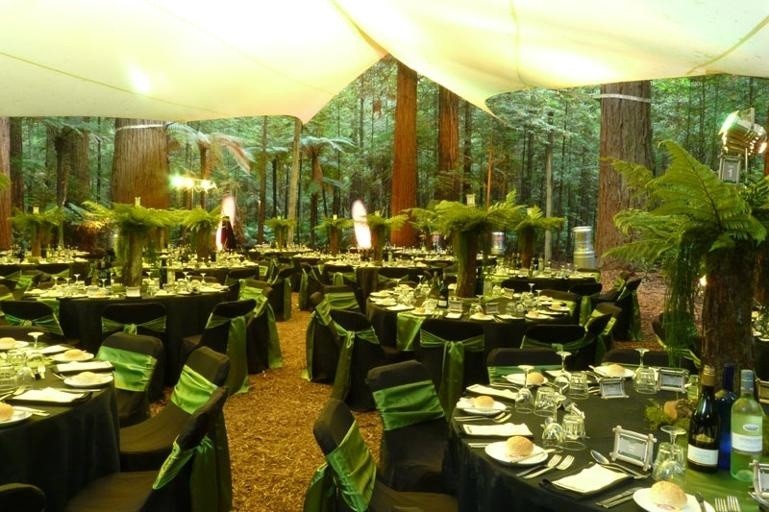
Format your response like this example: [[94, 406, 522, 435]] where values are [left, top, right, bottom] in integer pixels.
[[605, 363, 628, 376], [0, 337, 17, 351], [528, 372, 544, 387], [77, 371, 96, 384], [528, 309, 537, 318], [504, 435, 535, 457], [64, 348, 86, 363], [1, 403, 14, 422], [651, 480, 687, 511], [474, 396, 495, 413]]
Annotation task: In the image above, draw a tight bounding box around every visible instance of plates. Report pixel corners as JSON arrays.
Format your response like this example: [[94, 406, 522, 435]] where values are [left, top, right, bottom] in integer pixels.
[[0, 408, 31, 426], [630, 486, 717, 512], [485, 441, 548, 465], [458, 400, 507, 416], [593, 365, 635, 380], [64, 374, 114, 388], [52, 351, 93, 363]]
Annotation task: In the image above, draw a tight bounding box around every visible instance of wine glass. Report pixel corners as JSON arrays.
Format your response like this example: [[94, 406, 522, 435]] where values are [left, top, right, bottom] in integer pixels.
[[632, 347, 650, 387], [552, 351, 572, 392], [651, 424, 687, 483], [52, 269, 209, 294], [513, 363, 535, 415], [26, 331, 46, 374], [378, 270, 547, 312]]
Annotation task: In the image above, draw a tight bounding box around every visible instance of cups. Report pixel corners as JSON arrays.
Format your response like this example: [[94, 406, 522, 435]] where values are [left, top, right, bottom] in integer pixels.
[[567, 372, 590, 400], [535, 387, 558, 420], [559, 417, 588, 450], [635, 368, 658, 395]]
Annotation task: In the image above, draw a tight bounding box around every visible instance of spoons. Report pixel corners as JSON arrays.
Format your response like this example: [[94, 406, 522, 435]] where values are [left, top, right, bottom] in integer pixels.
[[589, 450, 647, 482]]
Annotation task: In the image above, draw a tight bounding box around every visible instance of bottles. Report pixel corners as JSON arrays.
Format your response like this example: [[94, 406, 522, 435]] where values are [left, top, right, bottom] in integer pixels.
[[715, 363, 737, 475], [687, 363, 721, 473], [730, 369, 764, 484]]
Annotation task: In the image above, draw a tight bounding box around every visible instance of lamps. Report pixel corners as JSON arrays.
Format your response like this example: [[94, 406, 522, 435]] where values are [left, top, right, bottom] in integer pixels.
[[719, 108, 767, 182]]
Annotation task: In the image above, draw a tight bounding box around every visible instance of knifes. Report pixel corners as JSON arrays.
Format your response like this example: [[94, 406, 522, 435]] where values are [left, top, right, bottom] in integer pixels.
[[695, 491, 707, 512]]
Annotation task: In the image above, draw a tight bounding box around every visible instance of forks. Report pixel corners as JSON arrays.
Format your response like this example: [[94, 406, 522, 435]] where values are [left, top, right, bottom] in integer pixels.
[[518, 452, 562, 476], [715, 495, 742, 512], [525, 454, 577, 481]]
